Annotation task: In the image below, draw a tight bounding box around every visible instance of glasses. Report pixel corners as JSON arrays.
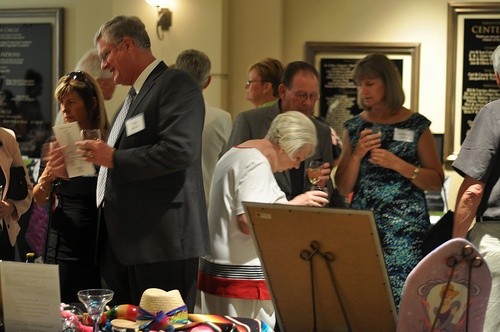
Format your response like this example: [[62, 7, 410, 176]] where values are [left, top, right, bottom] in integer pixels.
[[244, 80, 264, 88], [70, 72, 94, 93]]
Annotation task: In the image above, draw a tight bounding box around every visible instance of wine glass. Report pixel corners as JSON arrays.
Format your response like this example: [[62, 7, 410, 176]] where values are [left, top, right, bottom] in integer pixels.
[[80, 129, 100, 176], [61, 306, 84, 331], [310, 187, 328, 208], [77, 289, 114, 332], [309, 161, 324, 184], [366, 128, 381, 150]]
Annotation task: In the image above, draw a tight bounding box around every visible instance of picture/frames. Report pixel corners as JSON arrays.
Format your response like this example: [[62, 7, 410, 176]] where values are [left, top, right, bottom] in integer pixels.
[[394, 237, 494, 332], [241, 200, 397, 332]]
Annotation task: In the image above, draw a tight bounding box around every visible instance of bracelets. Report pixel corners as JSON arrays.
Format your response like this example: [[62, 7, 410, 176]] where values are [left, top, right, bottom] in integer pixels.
[[408, 166, 421, 182]]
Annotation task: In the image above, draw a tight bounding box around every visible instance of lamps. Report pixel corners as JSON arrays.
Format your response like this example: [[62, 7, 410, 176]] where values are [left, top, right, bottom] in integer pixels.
[[144, 0, 176, 40]]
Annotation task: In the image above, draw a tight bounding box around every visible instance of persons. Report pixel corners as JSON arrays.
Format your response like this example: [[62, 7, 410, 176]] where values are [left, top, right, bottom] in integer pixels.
[[242, 57, 284, 106], [451, 44, 499, 332], [334, 53, 447, 317], [202, 110, 330, 331], [33, 13, 233, 316], [0, 126, 34, 261], [221, 62, 339, 209]]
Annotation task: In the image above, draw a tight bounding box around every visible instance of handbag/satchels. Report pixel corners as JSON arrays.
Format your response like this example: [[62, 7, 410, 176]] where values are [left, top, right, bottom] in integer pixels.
[[420, 210, 453, 254], [7, 166, 28, 201]]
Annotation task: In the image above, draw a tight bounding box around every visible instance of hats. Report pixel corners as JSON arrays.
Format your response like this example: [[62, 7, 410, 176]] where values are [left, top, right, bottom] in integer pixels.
[[110, 288, 205, 331]]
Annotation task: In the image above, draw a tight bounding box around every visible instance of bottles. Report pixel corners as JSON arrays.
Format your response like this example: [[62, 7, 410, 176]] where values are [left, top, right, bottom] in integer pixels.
[[26, 252, 35, 263]]
